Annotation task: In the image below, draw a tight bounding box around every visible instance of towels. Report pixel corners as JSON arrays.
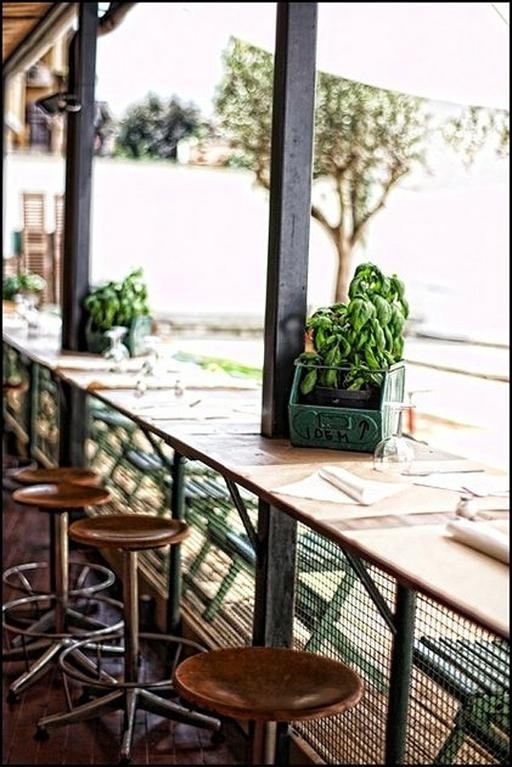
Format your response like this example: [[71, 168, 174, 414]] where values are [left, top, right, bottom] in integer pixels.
[[269, 457, 511, 566]]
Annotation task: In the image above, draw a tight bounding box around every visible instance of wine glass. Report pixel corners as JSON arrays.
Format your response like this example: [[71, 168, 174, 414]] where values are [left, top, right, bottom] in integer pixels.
[[374, 401, 417, 474], [103, 327, 130, 372]]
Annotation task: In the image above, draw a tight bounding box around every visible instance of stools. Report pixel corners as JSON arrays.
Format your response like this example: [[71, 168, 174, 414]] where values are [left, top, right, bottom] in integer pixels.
[[172, 644, 360, 766], [2, 483, 124, 697], [2, 469, 118, 603], [40, 511, 226, 753]]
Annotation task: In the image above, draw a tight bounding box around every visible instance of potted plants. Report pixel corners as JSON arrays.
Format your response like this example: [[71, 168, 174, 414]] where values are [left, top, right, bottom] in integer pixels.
[[1, 272, 47, 319], [82, 264, 151, 358], [289, 263, 411, 452]]
